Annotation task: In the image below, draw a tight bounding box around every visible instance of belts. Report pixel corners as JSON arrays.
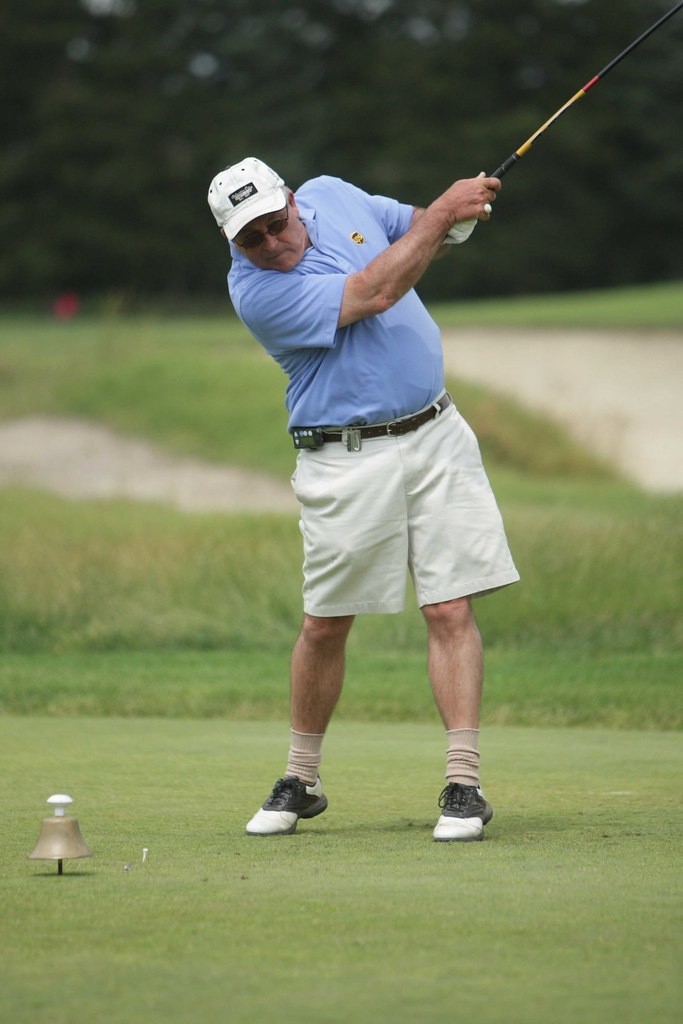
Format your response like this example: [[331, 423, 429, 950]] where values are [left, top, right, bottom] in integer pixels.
[[322, 393, 451, 442]]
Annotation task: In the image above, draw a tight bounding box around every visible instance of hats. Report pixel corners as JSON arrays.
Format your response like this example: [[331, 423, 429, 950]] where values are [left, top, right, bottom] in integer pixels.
[[208, 157, 286, 241]]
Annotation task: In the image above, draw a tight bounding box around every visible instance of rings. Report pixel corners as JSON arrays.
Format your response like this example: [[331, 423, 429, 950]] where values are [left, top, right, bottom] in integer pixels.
[[484, 203, 492, 215]]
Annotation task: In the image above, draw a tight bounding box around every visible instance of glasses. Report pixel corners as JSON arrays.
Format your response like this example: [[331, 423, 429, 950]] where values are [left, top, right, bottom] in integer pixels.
[[234, 200, 289, 249]]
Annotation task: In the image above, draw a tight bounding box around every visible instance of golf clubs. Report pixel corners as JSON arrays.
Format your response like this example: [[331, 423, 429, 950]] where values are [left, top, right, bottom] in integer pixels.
[[442, 0, 683, 246]]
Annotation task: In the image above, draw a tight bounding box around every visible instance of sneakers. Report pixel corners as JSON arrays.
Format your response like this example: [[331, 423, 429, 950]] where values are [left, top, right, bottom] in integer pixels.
[[432, 782, 493, 842], [245, 773, 328, 836]]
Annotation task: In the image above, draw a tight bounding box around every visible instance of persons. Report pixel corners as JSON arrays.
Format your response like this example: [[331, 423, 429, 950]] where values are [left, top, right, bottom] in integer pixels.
[[208, 158, 521, 842]]
